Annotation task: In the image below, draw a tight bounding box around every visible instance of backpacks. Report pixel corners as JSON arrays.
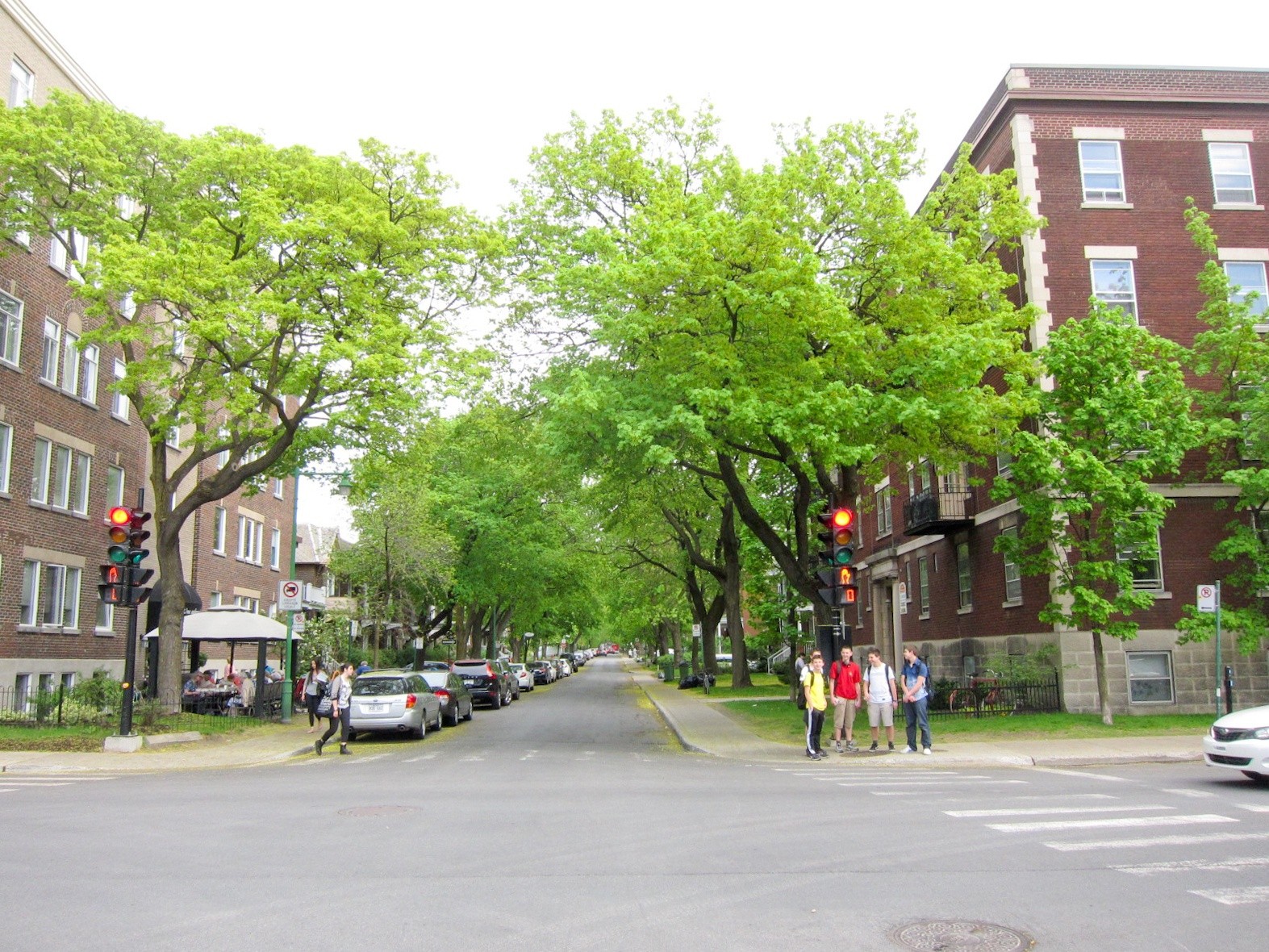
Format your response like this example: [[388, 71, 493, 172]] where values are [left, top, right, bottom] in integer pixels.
[[797, 670, 814, 709], [916, 662, 934, 702]]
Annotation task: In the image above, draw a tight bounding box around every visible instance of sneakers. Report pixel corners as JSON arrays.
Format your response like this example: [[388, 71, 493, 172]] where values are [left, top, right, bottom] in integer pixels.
[[815, 749, 829, 758], [868, 743, 879, 752], [888, 744, 896, 751], [900, 745, 913, 753], [806, 749, 820, 761], [923, 748, 931, 755], [845, 742, 858, 751], [834, 743, 843, 753]]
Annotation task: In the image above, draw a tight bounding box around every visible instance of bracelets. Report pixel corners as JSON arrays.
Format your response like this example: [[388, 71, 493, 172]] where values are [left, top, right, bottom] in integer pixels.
[[892, 699, 897, 703]]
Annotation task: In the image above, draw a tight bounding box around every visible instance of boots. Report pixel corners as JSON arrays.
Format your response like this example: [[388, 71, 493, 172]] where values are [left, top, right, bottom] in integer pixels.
[[339, 744, 351, 754], [315, 739, 323, 755]]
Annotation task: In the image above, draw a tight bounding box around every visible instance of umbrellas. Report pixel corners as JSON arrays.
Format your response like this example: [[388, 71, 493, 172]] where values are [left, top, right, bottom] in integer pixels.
[[140, 605, 307, 674]]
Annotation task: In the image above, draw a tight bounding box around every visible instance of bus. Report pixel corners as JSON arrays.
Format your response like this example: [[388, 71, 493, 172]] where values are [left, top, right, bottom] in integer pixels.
[[597, 643, 619, 657]]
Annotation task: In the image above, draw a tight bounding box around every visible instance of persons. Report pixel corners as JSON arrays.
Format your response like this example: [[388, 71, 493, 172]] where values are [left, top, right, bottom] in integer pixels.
[[183, 656, 285, 716], [897, 643, 934, 756], [794, 652, 807, 710], [802, 655, 829, 762], [829, 645, 863, 754], [858, 644, 899, 754], [799, 648, 825, 682], [314, 662, 356, 756], [355, 659, 373, 676], [300, 657, 328, 735]]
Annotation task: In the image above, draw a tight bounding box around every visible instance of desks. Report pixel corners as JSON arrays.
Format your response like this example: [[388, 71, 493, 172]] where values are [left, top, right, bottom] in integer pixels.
[[184, 690, 240, 719]]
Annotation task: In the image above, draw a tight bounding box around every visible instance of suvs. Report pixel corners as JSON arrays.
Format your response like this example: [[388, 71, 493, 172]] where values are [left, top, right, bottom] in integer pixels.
[[490, 659, 521, 699], [447, 659, 512, 708], [560, 652, 577, 672]]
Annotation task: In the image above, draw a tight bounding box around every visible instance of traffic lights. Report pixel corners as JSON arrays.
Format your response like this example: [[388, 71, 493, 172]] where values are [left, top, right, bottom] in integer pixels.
[[100, 505, 153, 604], [818, 505, 857, 604]]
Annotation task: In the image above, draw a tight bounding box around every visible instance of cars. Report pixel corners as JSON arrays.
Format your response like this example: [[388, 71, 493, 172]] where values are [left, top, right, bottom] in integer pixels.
[[579, 648, 599, 661], [547, 659, 564, 679], [1202, 700, 1269, 786], [560, 659, 574, 676], [574, 654, 584, 666], [403, 661, 449, 674], [574, 651, 587, 662], [414, 670, 475, 725], [526, 663, 552, 685], [507, 663, 536, 692], [348, 668, 443, 737], [534, 660, 557, 681]]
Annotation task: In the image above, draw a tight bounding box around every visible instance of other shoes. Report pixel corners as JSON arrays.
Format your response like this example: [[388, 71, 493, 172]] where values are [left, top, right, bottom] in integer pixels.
[[307, 723, 322, 733]]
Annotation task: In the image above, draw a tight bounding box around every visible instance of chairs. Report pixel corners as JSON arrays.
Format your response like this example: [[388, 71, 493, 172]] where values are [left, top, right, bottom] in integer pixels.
[[231, 680, 283, 716]]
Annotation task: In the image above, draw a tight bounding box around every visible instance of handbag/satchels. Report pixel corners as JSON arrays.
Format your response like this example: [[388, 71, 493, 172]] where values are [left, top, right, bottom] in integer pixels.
[[796, 665, 802, 674], [316, 695, 334, 718]]
